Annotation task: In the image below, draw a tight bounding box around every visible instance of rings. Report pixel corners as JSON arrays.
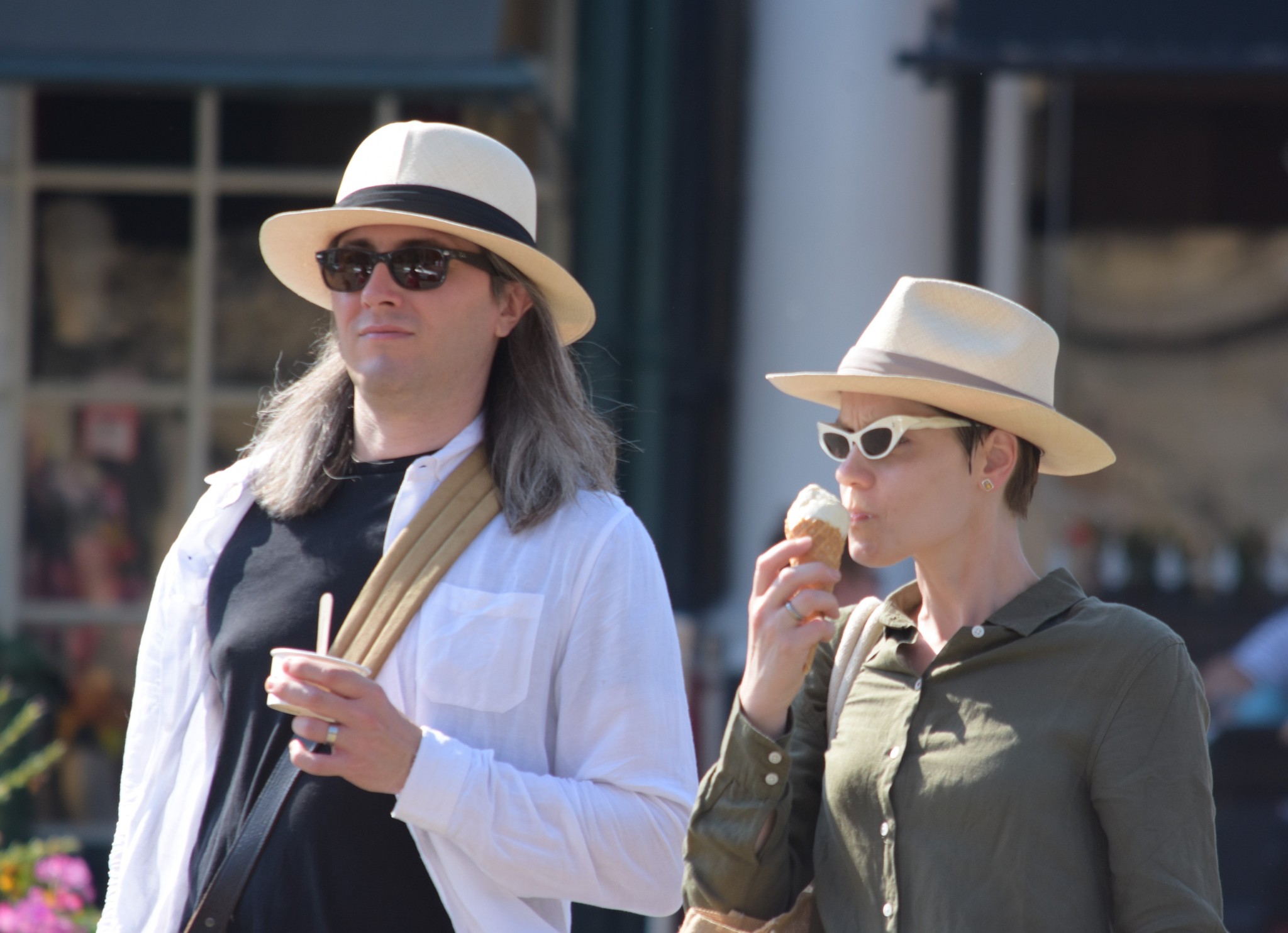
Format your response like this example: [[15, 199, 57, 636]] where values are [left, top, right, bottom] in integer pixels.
[[784, 601, 804, 623], [324, 725, 338, 747]]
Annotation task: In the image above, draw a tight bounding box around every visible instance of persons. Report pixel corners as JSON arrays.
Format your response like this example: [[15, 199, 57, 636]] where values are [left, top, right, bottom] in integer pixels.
[[682, 276, 1229, 933], [95, 120, 698, 933]]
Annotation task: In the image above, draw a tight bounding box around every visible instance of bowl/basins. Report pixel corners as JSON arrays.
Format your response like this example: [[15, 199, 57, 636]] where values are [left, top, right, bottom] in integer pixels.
[[267, 648, 372, 723]]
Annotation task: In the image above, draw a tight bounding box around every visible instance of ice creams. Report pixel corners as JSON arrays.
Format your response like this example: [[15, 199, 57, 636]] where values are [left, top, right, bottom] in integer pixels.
[[783, 483, 851, 673]]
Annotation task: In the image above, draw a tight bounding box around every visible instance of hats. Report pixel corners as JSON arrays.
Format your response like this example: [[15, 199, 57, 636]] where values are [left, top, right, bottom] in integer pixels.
[[765, 276, 1116, 476], [259, 120, 596, 345]]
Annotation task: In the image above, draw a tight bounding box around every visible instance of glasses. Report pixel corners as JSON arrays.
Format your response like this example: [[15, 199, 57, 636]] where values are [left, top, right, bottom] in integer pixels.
[[314, 245, 497, 291], [817, 416, 982, 462]]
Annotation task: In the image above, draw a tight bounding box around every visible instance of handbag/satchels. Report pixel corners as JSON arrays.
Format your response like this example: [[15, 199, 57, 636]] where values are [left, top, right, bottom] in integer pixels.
[[680, 595, 884, 933]]
